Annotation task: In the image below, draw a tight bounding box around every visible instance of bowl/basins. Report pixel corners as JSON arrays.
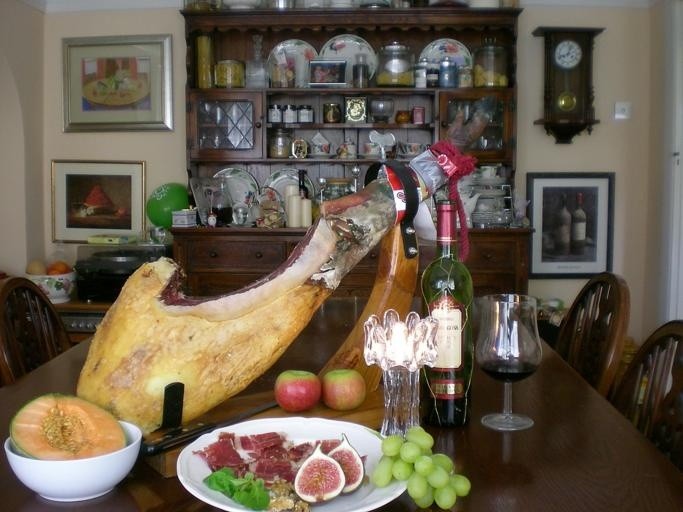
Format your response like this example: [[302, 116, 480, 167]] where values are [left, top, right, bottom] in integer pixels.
[[4, 420, 142, 503], [481, 166, 497, 176], [24, 269, 77, 304]]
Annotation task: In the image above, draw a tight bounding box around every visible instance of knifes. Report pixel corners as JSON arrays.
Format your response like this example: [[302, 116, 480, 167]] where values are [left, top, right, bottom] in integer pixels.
[[141, 400, 278, 457]]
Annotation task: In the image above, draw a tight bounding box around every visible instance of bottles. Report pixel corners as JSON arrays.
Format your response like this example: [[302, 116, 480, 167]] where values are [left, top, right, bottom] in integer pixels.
[[212, 173, 233, 225], [214, 60, 245, 88], [269, 129, 291, 159], [198, 34, 212, 87], [245, 34, 271, 87], [554, 194, 571, 255], [421, 200, 474, 428], [298, 171, 308, 199], [315, 178, 354, 205], [473, 37, 509, 88], [571, 192, 586, 255], [269, 53, 473, 86], [377, 40, 415, 86], [268, 103, 341, 123]]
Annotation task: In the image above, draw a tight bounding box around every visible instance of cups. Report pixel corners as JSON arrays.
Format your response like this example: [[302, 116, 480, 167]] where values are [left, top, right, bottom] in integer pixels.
[[364, 142, 380, 153], [285, 185, 312, 228], [404, 142, 428, 154], [395, 109, 411, 124]]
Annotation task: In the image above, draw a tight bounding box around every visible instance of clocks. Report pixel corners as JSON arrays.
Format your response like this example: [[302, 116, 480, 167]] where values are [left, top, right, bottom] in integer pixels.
[[530, 26, 605, 144]]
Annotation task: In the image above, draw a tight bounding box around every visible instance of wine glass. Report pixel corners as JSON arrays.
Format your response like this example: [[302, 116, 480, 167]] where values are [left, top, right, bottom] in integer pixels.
[[475, 294, 542, 431]]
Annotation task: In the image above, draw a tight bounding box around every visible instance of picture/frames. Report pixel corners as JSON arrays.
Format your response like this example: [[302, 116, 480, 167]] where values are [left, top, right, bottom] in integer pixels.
[[526, 170, 616, 280], [50, 158, 146, 247], [61, 33, 175, 134], [309, 60, 347, 87]]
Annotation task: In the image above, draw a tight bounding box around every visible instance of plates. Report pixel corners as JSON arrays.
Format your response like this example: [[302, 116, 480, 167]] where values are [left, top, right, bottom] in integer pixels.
[[397, 154, 419, 160], [356, 152, 389, 160], [176, 416, 408, 512], [319, 34, 375, 86], [308, 153, 335, 159], [267, 39, 317, 82], [422, 38, 472, 86], [262, 168, 314, 219], [472, 177, 512, 224], [212, 167, 259, 209]]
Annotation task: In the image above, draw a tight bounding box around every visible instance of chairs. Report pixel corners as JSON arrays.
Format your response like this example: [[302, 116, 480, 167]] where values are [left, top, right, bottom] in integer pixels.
[[610, 319, 682, 469], [0, 276, 71, 385], [550, 270, 630, 395]]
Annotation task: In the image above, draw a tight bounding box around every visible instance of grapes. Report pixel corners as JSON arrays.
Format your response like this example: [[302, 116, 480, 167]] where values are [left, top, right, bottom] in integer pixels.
[[373, 427, 471, 509]]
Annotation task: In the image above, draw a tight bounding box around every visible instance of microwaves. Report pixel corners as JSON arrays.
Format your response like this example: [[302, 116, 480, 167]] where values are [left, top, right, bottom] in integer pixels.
[[74, 243, 171, 304]]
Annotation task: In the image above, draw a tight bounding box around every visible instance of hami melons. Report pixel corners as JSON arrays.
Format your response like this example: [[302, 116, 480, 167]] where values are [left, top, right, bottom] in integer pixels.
[[9, 391, 127, 460]]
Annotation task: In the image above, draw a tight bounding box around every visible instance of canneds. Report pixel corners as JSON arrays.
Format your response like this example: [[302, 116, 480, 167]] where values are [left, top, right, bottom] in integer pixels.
[[426, 64, 441, 88], [268, 105, 314, 123]]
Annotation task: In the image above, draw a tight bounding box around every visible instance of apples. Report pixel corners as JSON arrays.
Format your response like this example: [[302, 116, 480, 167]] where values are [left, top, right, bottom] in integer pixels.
[[321, 369, 367, 412], [274, 370, 321, 413]]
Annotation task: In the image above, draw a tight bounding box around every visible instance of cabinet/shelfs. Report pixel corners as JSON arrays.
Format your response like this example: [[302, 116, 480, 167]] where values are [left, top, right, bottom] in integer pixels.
[[171, 9, 535, 302]]
